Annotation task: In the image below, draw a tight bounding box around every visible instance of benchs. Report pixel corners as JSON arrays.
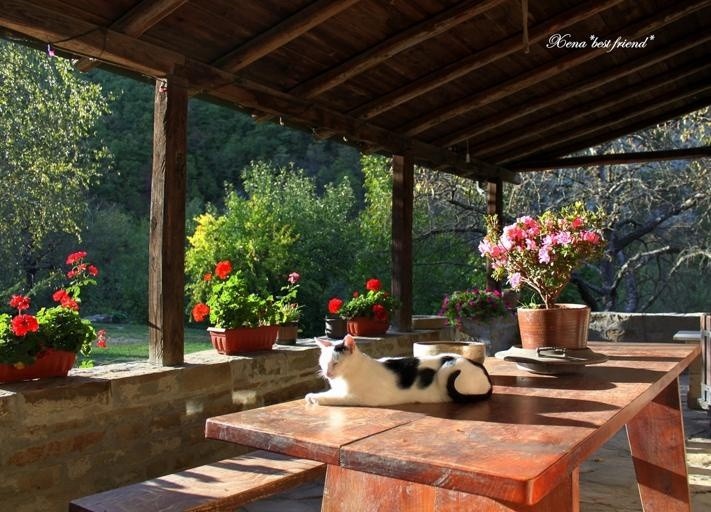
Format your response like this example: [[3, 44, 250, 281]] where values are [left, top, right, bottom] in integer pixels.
[[68, 449, 329, 512], [589, 307, 709, 412]]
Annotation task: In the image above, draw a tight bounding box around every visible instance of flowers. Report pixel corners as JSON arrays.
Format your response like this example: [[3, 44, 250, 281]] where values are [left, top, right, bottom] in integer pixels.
[[0, 245, 107, 360], [328, 277, 400, 317], [431, 287, 504, 326], [478, 202, 621, 303], [274, 271, 307, 319], [185, 257, 273, 326]]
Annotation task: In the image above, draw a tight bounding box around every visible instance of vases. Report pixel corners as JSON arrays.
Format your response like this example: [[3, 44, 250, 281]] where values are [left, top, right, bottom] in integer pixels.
[[511, 300, 593, 354], [345, 316, 392, 339], [279, 319, 300, 343], [206, 324, 278, 358], [0, 350, 82, 384], [323, 316, 346, 339]]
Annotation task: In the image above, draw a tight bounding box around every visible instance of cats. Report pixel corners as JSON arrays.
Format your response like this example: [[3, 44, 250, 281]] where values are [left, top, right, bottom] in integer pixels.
[[304, 331, 493, 408]]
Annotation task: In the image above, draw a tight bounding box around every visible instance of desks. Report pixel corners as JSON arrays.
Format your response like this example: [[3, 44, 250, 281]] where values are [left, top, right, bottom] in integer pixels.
[[196, 337, 701, 512]]
